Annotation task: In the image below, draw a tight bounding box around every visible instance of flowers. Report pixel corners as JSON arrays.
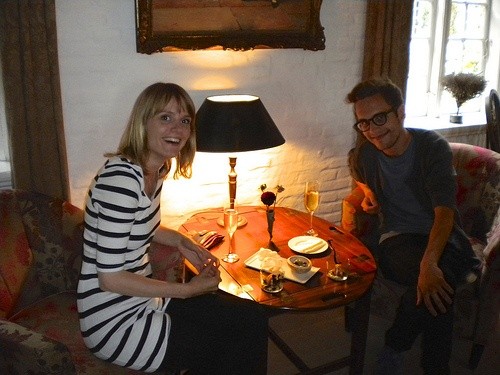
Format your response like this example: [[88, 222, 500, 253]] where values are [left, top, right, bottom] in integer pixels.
[[260, 184, 285, 212]]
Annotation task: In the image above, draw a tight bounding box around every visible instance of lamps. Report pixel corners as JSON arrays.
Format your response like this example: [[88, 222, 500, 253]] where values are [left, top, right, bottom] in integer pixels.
[[196, 94, 285, 227]]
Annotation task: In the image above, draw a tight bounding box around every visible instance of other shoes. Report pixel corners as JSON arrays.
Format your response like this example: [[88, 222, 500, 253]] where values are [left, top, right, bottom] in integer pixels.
[[423, 364, 450, 375], [376, 343, 401, 375]]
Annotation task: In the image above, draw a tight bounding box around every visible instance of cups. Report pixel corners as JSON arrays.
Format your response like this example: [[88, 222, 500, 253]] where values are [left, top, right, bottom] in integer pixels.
[[325, 255, 351, 281], [258, 256, 284, 293]]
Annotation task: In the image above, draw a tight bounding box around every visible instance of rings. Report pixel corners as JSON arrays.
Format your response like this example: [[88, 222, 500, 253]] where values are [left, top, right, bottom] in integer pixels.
[[201, 265, 204, 267]]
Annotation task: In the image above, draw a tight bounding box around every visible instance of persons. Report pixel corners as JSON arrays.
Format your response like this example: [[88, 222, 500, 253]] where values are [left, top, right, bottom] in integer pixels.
[[344, 75, 472, 375], [77, 82, 268, 375]]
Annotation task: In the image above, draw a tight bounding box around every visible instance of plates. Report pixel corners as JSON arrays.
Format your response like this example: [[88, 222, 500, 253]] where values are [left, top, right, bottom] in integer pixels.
[[243, 248, 321, 284], [287, 235, 328, 254]]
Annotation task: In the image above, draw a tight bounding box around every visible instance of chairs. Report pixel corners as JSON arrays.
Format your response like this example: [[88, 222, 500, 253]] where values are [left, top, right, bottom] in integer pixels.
[[485, 89, 500, 154], [341, 142, 500, 369], [0, 188, 185, 375]]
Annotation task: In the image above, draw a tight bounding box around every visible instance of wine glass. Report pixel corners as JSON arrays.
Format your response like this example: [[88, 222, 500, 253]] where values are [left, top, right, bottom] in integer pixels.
[[222, 198, 240, 263], [305, 179, 319, 236]]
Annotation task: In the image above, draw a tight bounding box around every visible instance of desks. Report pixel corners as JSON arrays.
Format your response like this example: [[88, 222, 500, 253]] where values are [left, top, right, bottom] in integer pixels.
[[178, 205, 377, 375]]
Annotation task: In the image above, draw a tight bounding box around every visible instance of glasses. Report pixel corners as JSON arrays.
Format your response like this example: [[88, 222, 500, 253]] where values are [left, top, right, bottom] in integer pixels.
[[353, 106, 398, 132]]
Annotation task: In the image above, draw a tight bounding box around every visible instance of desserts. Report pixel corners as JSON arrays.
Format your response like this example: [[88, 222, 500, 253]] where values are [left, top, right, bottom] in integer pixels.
[[286, 256, 312, 274]]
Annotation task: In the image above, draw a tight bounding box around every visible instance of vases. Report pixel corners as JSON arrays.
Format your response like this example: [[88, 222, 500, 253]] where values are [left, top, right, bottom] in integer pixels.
[[266, 210, 281, 252]]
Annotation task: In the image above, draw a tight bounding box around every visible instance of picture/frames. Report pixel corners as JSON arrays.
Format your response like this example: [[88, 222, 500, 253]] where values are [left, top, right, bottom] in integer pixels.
[[134, 0, 326, 55]]
